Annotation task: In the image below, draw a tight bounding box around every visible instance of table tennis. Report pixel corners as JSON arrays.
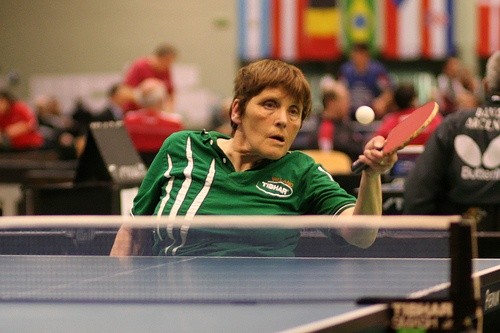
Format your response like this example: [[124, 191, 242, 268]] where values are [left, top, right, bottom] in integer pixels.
[[355, 106, 375, 124]]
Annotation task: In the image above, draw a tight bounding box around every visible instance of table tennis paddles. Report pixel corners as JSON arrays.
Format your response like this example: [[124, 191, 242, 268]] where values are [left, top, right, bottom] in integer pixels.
[[351, 101, 439, 174]]
